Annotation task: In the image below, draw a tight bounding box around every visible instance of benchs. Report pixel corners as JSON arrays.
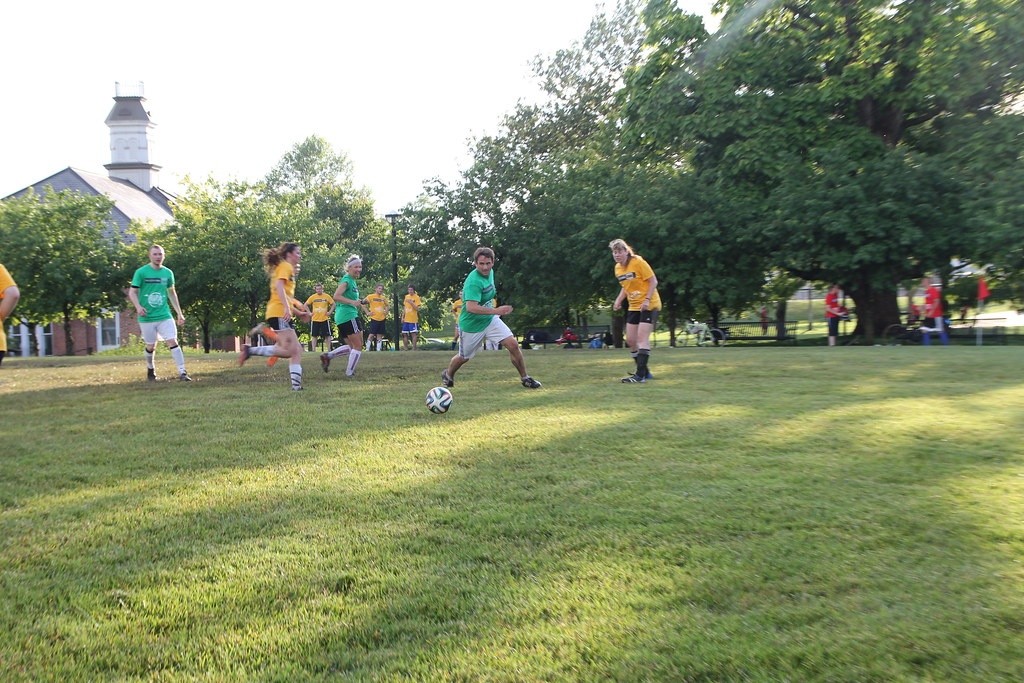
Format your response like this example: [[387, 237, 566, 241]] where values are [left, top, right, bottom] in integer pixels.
[[703, 322, 799, 345], [908, 319, 976, 344], [524, 325, 610, 348]]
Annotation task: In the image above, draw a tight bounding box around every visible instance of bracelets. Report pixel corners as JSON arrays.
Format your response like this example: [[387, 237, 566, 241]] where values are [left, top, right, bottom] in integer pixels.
[[645, 297, 650, 301]]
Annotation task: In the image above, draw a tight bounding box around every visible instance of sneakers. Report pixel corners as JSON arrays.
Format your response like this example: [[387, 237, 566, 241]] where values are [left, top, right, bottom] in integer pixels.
[[442, 369, 454, 387], [521, 377, 541, 388]]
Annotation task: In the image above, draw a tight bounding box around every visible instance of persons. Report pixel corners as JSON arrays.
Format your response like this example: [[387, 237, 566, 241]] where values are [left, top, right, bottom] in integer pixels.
[[761, 305, 769, 335], [129, 244, 192, 381], [441, 248, 541, 388], [240, 242, 313, 391], [825, 283, 846, 347], [361, 284, 390, 351], [303, 284, 336, 353], [609, 239, 662, 383], [320, 254, 363, 377], [0, 264, 20, 365], [910, 276, 949, 346], [451, 291, 499, 351], [402, 285, 421, 350]]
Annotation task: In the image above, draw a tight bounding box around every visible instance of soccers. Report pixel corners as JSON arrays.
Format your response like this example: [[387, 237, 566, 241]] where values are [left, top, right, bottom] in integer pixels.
[[423, 386, 455, 414]]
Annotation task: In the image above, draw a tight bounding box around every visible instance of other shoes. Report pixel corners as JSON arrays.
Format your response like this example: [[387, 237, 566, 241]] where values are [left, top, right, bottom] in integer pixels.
[[320, 353, 329, 373], [148, 368, 157, 380], [240, 344, 249, 367], [621, 372, 645, 383], [180, 373, 191, 381], [249, 323, 265, 335]]
